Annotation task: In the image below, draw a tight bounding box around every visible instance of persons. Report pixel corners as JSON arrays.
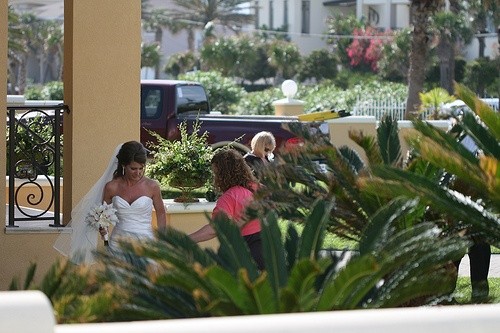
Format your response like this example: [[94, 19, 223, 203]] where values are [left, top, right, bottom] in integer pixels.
[[242, 130, 276, 197], [186, 149, 266, 278], [97, 140, 167, 287]]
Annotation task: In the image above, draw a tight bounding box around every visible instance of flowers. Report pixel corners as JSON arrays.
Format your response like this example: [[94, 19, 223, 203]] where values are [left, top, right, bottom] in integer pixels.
[[85, 201, 120, 246]]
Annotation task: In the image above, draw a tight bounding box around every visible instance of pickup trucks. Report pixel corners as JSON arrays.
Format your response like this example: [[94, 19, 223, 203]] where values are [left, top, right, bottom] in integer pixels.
[[54, 79, 330, 167]]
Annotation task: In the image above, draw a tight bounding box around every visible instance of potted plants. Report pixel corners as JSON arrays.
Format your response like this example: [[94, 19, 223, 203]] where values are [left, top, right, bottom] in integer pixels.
[[143, 109, 246, 203]]
